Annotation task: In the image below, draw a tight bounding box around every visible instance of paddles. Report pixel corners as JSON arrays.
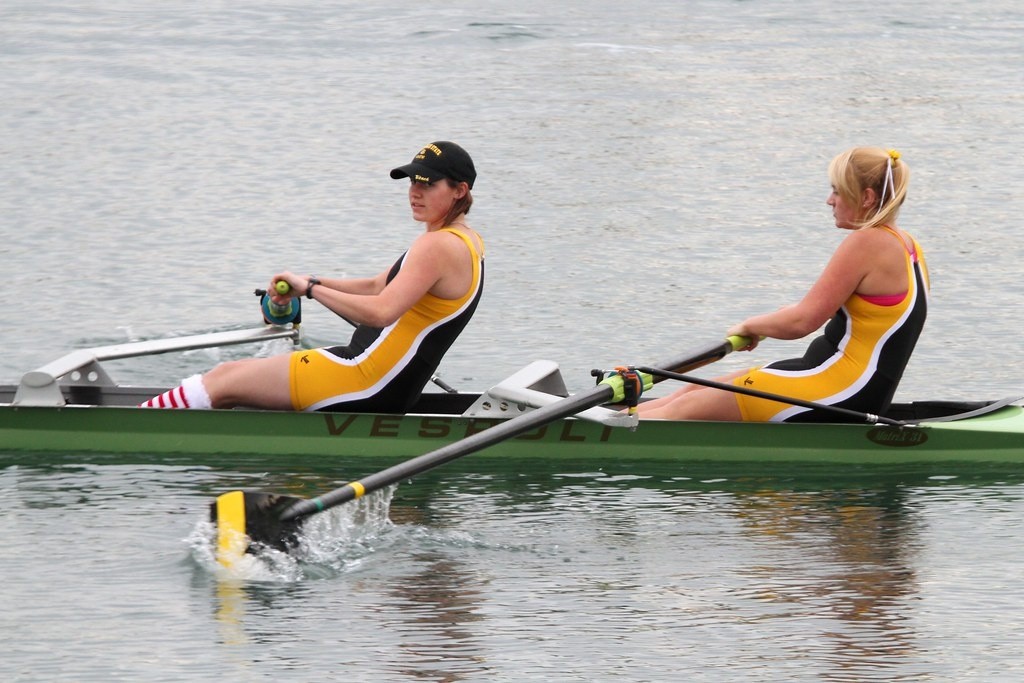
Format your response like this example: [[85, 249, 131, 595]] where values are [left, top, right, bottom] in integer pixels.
[[251, 280, 305, 332], [209, 332, 770, 571]]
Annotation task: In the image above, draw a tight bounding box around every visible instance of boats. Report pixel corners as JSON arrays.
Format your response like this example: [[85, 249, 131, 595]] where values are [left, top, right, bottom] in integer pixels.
[[0, 325, 1024, 460]]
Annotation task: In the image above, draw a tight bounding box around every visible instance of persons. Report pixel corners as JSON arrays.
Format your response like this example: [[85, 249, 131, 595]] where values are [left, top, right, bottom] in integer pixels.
[[620, 147, 930, 420], [135, 141, 484, 409]]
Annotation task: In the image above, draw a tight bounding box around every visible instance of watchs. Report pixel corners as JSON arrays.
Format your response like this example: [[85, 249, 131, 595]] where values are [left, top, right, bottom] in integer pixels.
[[307, 278, 321, 299]]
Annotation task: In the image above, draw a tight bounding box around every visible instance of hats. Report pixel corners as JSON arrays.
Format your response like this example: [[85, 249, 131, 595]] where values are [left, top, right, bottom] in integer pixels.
[[390, 141, 477, 190]]
